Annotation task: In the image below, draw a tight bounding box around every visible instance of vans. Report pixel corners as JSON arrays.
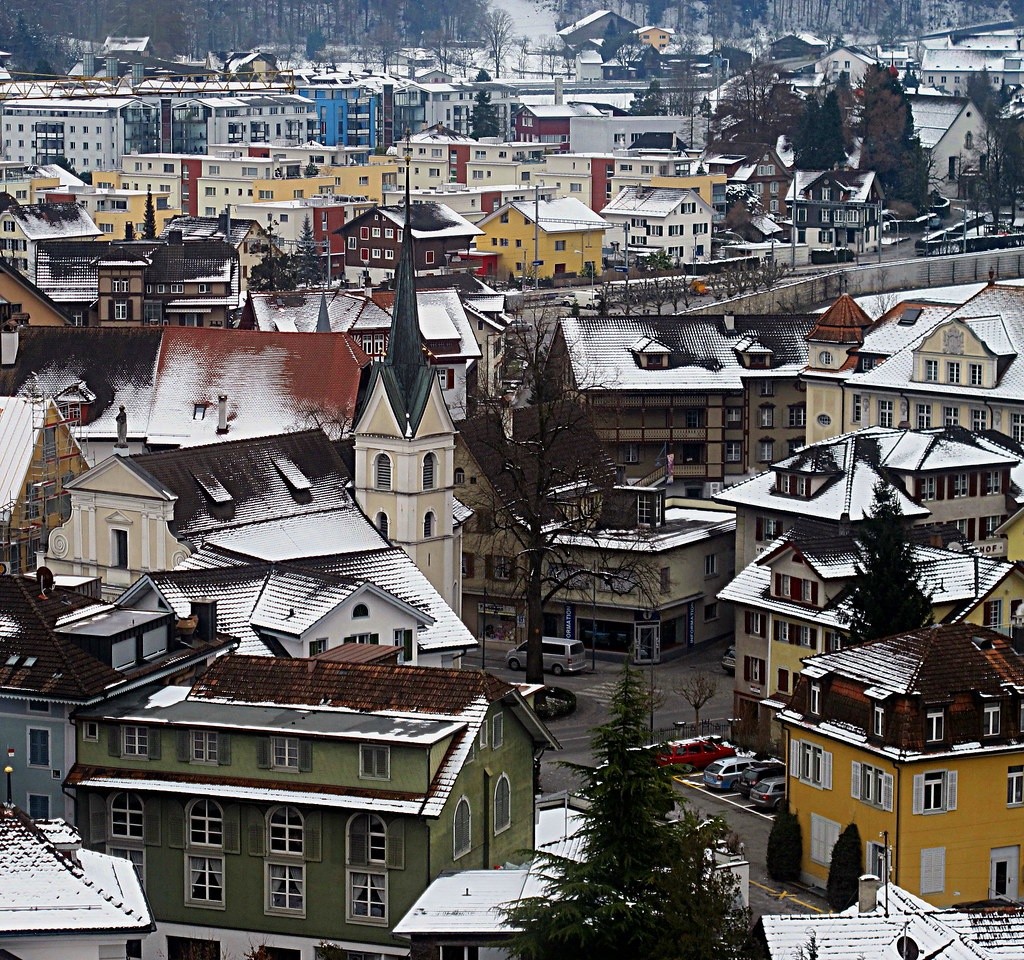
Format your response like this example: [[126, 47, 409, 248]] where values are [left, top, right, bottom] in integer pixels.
[[505, 637, 588, 676]]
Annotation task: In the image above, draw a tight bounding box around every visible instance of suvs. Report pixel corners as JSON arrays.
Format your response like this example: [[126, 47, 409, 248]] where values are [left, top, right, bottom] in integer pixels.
[[748, 776, 785, 813], [739, 766, 786, 798], [655, 741, 737, 773]]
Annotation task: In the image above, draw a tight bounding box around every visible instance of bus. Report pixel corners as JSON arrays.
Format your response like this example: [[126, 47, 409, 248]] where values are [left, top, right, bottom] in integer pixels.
[[704, 757, 764, 793]]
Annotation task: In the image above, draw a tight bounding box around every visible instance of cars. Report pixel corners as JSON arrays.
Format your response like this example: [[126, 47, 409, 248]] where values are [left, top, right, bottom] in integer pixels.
[[721, 645, 736, 673], [535, 685, 577, 717]]
